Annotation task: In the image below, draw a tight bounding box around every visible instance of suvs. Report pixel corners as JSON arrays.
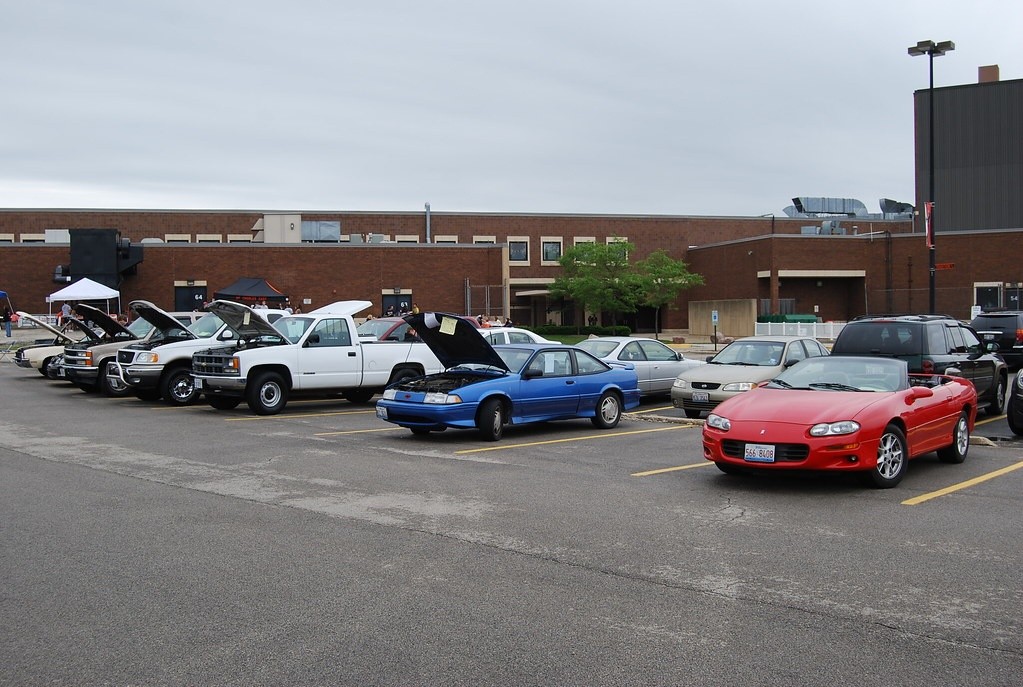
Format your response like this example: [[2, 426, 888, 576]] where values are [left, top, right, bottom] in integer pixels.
[[969, 307, 1023, 371], [829, 312, 1008, 417]]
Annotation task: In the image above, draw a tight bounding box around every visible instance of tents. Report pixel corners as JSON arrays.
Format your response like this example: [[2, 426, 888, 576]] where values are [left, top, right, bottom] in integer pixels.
[[0, 291, 14, 314], [50, 278, 120, 325], [213, 277, 289, 302]]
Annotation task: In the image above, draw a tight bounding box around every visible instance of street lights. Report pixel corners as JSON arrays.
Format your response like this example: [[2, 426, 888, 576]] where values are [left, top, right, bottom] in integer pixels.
[[907, 39, 957, 312]]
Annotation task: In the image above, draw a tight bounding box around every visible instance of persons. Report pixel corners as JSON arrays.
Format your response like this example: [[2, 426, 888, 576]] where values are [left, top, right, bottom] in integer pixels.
[[413, 303, 419, 314], [255, 300, 268, 309], [62, 301, 73, 333], [384, 305, 394, 317], [481, 318, 491, 328], [284, 304, 293, 315], [366, 315, 372, 321], [494, 316, 501, 323], [588, 315, 598, 326], [477, 313, 484, 324], [294, 304, 302, 314], [199, 302, 209, 312], [504, 318, 512, 327], [401, 304, 408, 312], [3, 305, 12, 337], [403, 325, 422, 342], [121, 312, 129, 326]]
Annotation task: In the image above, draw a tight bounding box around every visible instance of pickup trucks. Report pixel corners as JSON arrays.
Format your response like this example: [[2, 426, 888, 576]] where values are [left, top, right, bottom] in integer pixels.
[[188, 299, 446, 417]]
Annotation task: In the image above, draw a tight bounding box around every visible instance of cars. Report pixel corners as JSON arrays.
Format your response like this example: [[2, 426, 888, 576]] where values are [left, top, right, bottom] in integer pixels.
[[473, 327, 563, 359], [565, 336, 708, 398], [104, 299, 373, 406], [670, 335, 830, 419], [11, 304, 240, 398], [1007, 368, 1023, 437]]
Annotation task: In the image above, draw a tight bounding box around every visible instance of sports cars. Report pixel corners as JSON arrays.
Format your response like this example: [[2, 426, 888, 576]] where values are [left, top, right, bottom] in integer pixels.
[[374, 311, 642, 442], [702, 355, 978, 490]]
